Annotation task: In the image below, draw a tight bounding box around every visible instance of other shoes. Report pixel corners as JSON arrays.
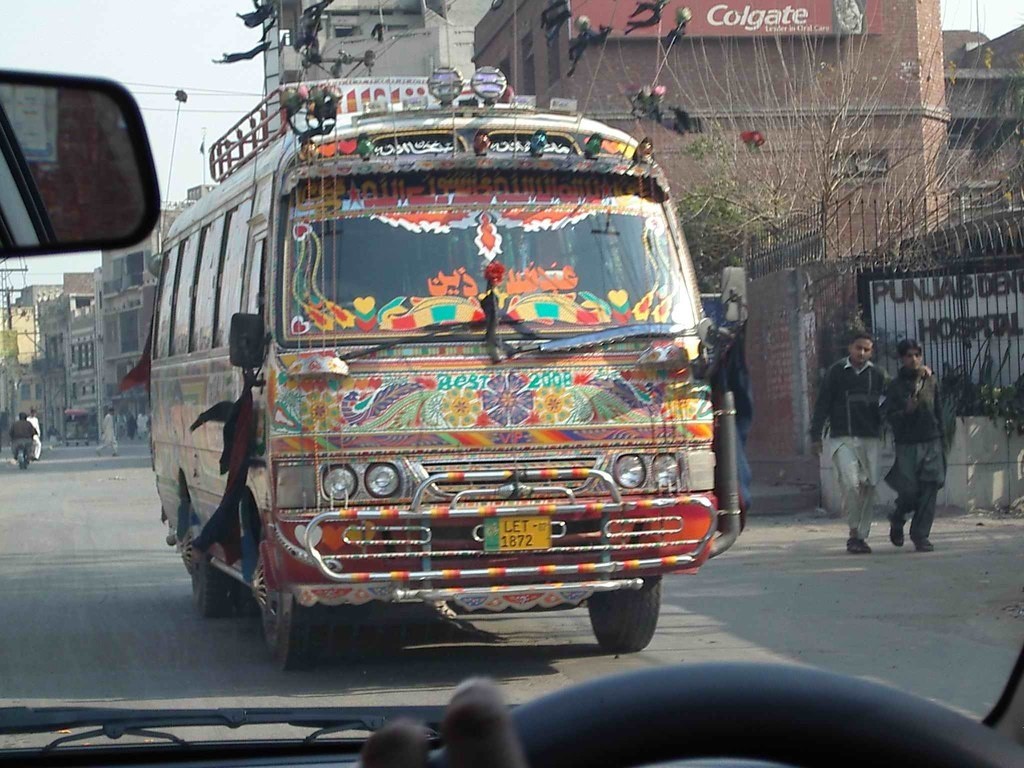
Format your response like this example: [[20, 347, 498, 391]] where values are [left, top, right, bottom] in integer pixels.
[[858, 539, 871, 552], [847, 538, 863, 553], [95, 449, 100, 457], [888, 511, 906, 546], [113, 452, 119, 457], [910, 533, 933, 552]]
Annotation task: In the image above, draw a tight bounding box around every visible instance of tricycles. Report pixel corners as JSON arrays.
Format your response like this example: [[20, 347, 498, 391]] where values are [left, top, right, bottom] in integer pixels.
[[64, 409, 89, 446]]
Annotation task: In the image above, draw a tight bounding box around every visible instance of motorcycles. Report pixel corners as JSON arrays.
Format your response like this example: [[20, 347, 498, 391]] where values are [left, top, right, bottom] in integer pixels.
[[16, 445, 29, 470]]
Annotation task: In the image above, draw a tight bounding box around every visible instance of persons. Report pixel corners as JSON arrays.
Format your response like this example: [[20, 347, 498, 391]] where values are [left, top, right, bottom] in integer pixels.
[[96, 408, 121, 456], [9, 408, 41, 464], [810, 332, 950, 555], [49, 424, 60, 450], [361, 676, 527, 768], [115, 410, 151, 443]]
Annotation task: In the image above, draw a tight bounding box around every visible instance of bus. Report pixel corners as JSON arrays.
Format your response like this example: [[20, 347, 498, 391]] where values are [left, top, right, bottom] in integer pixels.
[[147, 67, 748, 670]]
